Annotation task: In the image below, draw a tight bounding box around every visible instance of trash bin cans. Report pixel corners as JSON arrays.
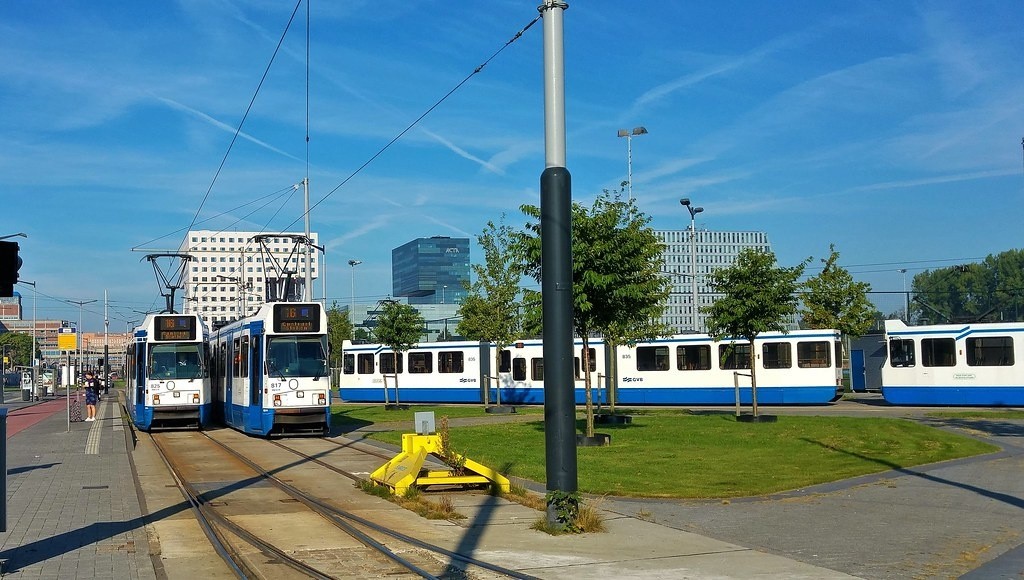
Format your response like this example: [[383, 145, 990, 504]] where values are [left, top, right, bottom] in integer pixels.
[[841, 379, 851, 392], [111, 383, 114, 388], [843, 368, 849, 378], [22, 389, 30, 400], [0, 407, 9, 532]]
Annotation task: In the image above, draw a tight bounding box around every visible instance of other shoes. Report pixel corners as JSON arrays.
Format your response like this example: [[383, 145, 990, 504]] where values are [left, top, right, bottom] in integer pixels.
[[85, 417, 95, 421]]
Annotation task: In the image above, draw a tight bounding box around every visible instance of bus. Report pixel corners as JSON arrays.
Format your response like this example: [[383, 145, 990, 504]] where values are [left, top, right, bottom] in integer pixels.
[[209, 232, 335, 441], [125, 254, 211, 433], [339, 327, 850, 409], [879, 319, 1024, 407]]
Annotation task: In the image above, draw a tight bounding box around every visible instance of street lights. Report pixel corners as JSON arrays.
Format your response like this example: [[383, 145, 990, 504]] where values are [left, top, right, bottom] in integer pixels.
[[347, 259, 362, 340], [63, 300, 98, 390], [2, 344, 12, 393], [443, 285, 448, 304]]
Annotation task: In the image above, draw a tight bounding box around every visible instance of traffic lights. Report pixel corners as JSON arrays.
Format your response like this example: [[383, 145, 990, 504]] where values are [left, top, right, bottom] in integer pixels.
[[617, 127, 648, 230], [898, 269, 908, 321], [680, 200, 704, 332]]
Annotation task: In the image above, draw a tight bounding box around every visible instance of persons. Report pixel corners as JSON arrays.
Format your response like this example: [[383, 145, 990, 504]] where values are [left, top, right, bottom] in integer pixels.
[[82, 372, 100, 421], [178, 355, 189, 366]]
